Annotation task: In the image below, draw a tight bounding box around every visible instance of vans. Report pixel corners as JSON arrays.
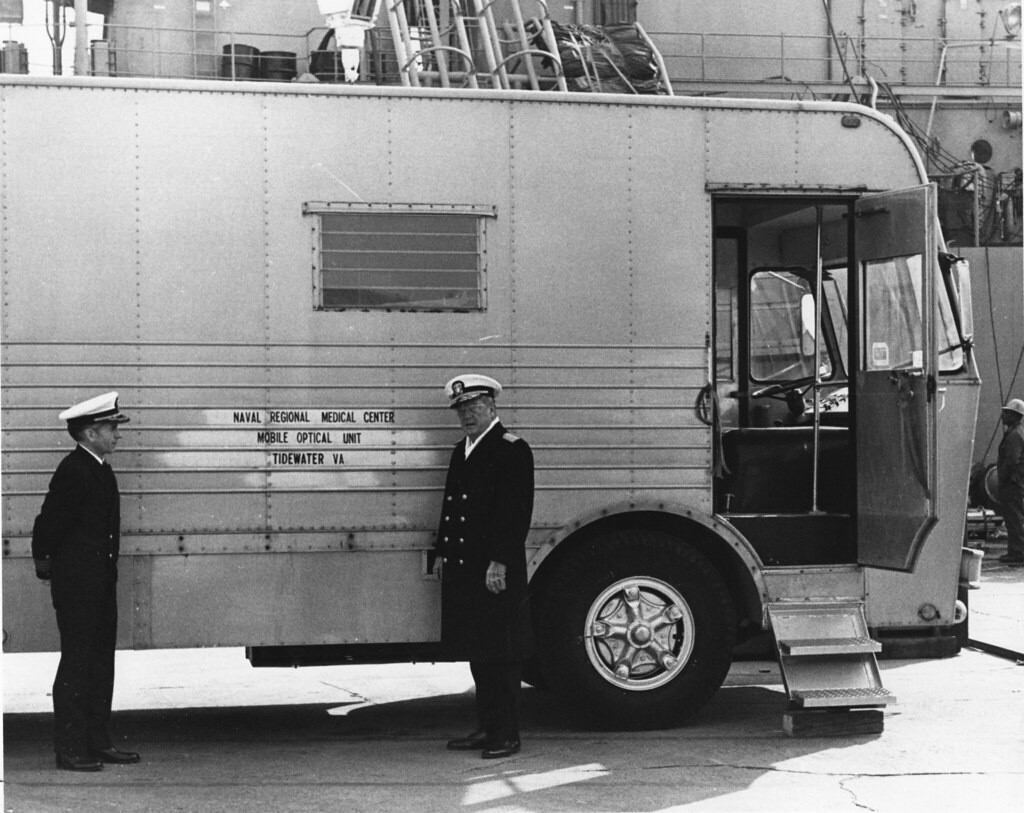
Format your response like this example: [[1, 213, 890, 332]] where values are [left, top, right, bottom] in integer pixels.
[[0, 70, 983, 734]]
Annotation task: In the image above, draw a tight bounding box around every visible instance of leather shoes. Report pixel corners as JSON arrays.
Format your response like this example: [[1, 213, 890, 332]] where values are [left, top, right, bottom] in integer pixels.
[[55, 745, 103, 771], [88, 747, 140, 763], [482, 738, 520, 758], [447, 731, 498, 751]]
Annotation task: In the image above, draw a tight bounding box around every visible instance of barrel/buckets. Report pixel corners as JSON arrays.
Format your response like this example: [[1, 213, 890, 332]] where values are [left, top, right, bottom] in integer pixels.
[[260, 51, 297, 79], [222, 44, 260, 78], [310, 50, 345, 82]]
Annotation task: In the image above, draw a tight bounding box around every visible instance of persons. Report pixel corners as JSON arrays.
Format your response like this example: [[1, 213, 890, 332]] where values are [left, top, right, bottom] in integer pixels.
[[31, 391, 139, 771], [433, 373, 535, 759], [997, 399, 1024, 564]]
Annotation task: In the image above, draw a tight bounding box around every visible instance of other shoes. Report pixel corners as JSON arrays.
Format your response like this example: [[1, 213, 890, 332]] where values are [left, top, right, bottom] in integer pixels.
[[999, 555, 1024, 565]]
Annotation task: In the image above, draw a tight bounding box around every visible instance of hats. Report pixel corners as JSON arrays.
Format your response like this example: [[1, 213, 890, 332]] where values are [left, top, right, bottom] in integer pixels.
[[444, 374, 502, 409], [58, 391, 131, 423]]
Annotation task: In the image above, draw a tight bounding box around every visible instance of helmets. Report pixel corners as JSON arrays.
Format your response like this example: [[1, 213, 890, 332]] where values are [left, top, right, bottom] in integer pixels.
[[1000, 398, 1024, 415]]
